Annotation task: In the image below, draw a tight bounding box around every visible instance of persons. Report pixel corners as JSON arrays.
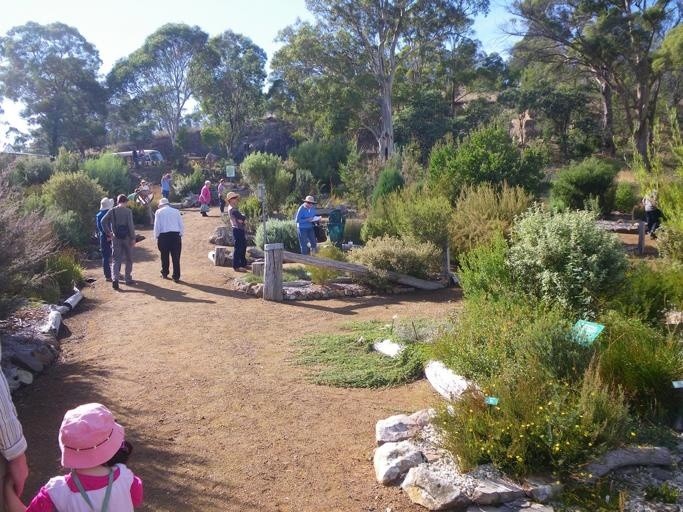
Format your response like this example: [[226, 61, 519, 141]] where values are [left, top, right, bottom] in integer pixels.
[[198, 180, 212, 217], [225, 192, 252, 273], [295, 196, 323, 255], [160, 174, 171, 200], [95, 197, 126, 282], [100, 194, 135, 290], [0, 363, 28, 512], [126, 179, 156, 226], [3, 402, 143, 512], [218, 178, 226, 216], [130, 147, 151, 168], [153, 198, 184, 283], [327, 203, 348, 252]]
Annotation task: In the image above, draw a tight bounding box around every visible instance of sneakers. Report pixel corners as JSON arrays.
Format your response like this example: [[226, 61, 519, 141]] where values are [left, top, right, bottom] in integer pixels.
[[236, 265, 252, 273], [106, 272, 135, 289], [200, 212, 208, 217], [171, 277, 179, 283], [160, 273, 168, 279]]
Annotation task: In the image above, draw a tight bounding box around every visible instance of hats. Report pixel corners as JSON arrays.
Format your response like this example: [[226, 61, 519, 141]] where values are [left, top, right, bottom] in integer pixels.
[[302, 195, 318, 204], [157, 198, 171, 208], [100, 197, 114, 211], [141, 179, 146, 183], [224, 192, 240, 203], [58, 402, 126, 470]]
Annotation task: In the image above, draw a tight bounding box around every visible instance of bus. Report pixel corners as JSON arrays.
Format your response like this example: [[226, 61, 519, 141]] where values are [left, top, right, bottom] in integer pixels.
[[110, 148, 164, 169]]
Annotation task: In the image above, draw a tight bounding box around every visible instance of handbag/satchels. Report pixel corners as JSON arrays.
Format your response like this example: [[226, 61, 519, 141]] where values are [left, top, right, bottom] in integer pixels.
[[200, 204, 210, 212], [314, 224, 327, 243], [116, 225, 128, 239]]
